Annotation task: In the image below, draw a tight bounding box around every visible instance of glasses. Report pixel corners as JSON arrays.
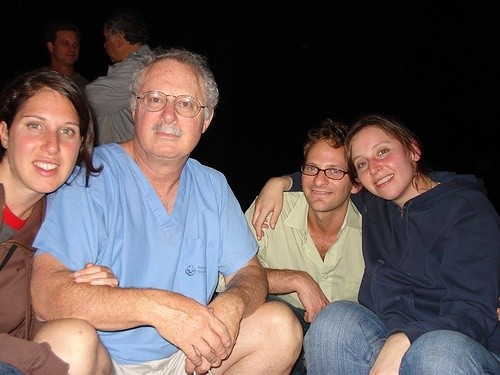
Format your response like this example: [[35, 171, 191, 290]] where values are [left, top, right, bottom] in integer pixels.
[[299, 163, 350, 180], [131, 89, 208, 117]]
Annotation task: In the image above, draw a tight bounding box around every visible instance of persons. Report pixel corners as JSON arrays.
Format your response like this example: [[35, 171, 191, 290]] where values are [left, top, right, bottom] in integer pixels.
[[30, 48, 304, 375], [0, 70, 119, 375], [244, 119, 366, 375], [253, 116, 500, 375], [48, 27, 88, 87], [84, 13, 152, 147]]
